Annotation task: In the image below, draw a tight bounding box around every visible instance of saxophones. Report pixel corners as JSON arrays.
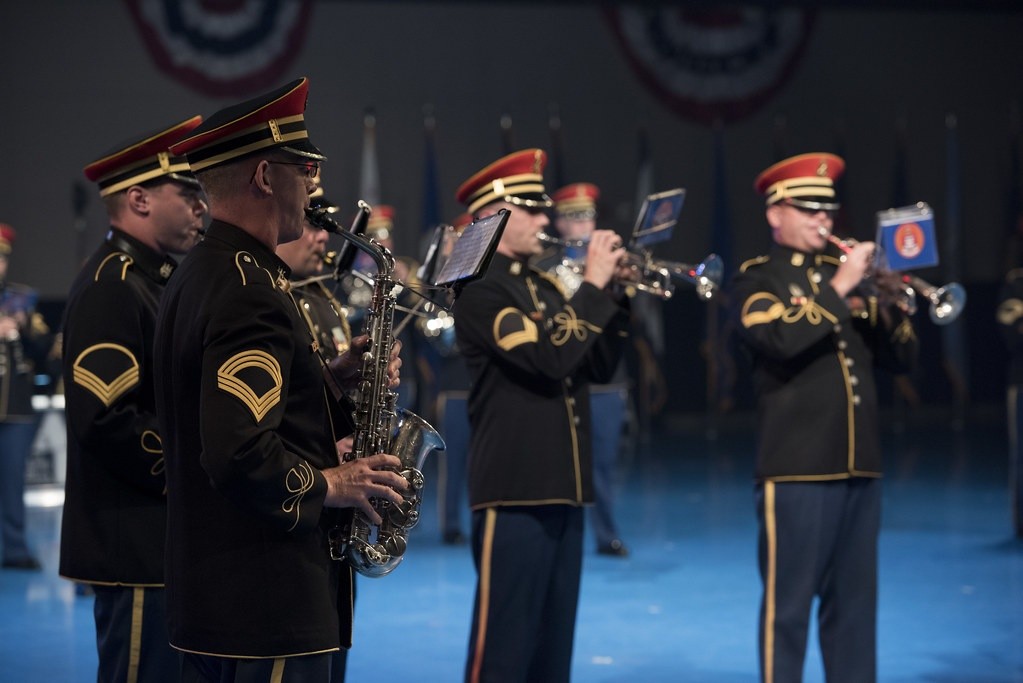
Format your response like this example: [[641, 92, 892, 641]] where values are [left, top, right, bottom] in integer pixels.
[[307, 205, 446, 577]]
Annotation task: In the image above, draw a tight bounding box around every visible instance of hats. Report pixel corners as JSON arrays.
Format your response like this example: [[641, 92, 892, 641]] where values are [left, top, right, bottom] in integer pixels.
[[170, 77, 328, 174], [1, 224, 19, 254], [756, 152, 846, 210], [458, 148, 556, 217], [309, 166, 340, 213], [366, 207, 398, 240], [84, 114, 203, 197], [552, 182, 601, 219]]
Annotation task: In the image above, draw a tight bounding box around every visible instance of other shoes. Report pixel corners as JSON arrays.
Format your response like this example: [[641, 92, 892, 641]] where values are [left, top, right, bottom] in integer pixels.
[[5, 558, 41, 572], [444, 528, 468, 546], [599, 541, 630, 558]]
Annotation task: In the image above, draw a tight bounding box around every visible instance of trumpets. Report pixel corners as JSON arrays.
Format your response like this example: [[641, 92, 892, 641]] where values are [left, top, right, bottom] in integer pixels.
[[536, 232, 723, 299], [819, 226, 964, 325]]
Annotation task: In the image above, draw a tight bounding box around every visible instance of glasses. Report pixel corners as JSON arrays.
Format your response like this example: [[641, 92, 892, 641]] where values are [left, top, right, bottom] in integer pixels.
[[250, 163, 319, 185]]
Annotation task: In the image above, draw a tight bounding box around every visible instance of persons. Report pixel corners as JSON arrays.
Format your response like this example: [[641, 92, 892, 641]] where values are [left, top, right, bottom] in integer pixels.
[[714, 153, 900, 683], [152, 75, 409, 683], [453, 147, 632, 683], [58, 110, 208, 683], [0, 178, 1023, 597]]
